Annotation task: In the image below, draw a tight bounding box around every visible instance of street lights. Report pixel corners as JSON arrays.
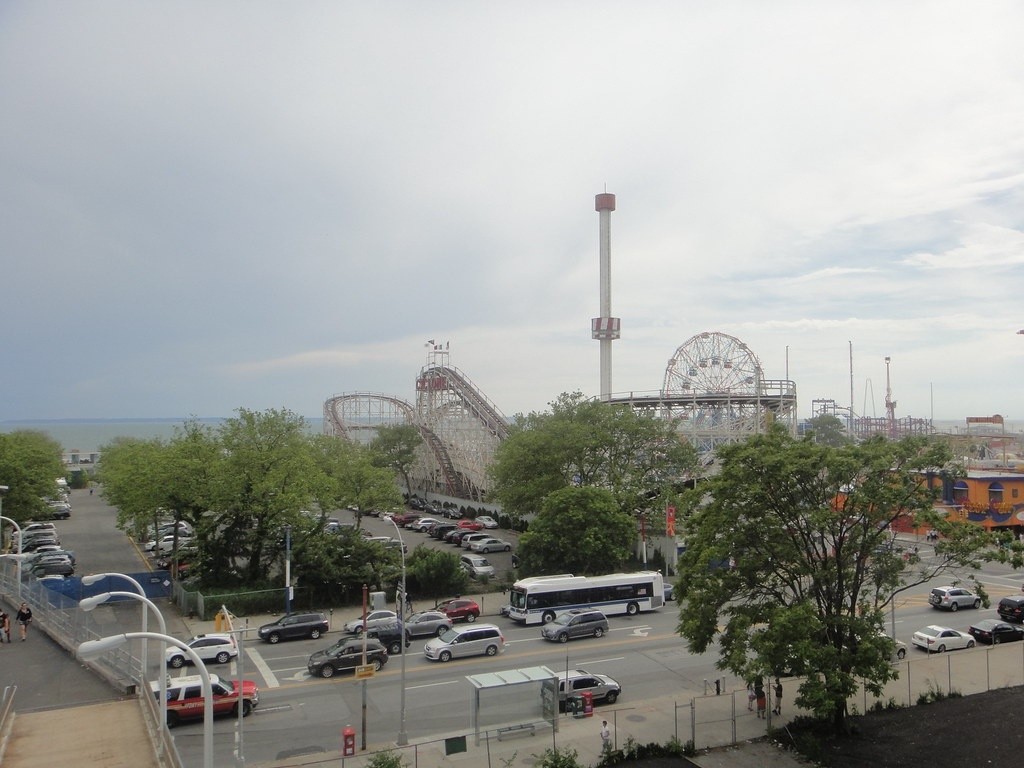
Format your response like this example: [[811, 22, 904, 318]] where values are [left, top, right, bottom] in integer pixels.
[[76, 572, 213, 768], [383, 515, 409, 746]]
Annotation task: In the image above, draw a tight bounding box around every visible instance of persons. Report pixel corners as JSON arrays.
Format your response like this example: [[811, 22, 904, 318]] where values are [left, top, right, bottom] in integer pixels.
[[598, 721, 610, 757], [754, 683, 766, 719], [755, 675, 764, 694], [772, 678, 782, 715], [926, 528, 939, 542], [15, 602, 32, 641], [0, 609, 11, 643], [747, 681, 757, 711]]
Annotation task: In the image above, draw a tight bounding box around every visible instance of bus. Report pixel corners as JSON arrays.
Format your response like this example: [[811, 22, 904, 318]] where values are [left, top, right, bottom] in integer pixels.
[[503, 569, 665, 626]]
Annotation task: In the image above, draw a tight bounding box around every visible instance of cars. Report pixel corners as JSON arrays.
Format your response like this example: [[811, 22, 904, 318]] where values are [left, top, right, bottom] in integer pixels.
[[298, 498, 513, 580], [662, 582, 677, 601], [911, 625, 977, 654], [343, 609, 397, 635], [144, 507, 199, 570], [968, 618, 1024, 645], [855, 631, 909, 661], [500, 598, 524, 617]]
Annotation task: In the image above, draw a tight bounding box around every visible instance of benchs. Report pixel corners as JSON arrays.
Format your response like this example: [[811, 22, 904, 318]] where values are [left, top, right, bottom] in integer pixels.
[[497, 723, 535, 741]]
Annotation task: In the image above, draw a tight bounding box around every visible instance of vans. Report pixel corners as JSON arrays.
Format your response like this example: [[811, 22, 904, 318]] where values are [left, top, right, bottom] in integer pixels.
[[336, 624, 411, 655]]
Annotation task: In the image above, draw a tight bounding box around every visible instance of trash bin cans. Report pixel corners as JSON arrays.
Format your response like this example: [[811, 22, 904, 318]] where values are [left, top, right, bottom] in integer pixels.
[[572, 696, 585, 719], [580, 692, 593, 717], [343, 728, 355, 756]]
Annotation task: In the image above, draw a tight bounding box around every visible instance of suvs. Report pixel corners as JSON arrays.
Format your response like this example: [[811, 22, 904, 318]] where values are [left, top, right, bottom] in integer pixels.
[[430, 598, 480, 623], [149, 673, 260, 729], [424, 623, 504, 662], [927, 586, 981, 612], [165, 633, 238, 669], [8, 476, 77, 583], [257, 610, 331, 644], [996, 595, 1024, 625], [404, 609, 453, 639], [539, 669, 621, 713], [540, 608, 609, 643], [308, 636, 388, 678]]
